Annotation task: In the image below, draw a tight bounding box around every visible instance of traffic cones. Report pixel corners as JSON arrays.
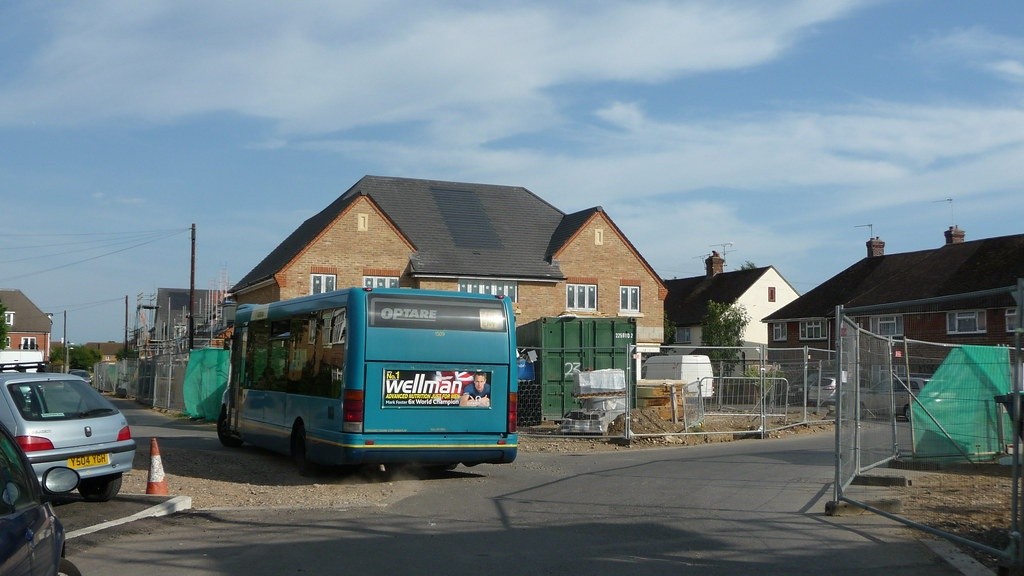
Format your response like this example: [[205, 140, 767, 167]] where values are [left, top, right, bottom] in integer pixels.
[[144, 437, 172, 495]]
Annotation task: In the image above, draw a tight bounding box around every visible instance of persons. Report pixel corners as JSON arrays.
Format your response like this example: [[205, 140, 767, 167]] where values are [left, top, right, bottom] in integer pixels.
[[459, 373, 491, 407]]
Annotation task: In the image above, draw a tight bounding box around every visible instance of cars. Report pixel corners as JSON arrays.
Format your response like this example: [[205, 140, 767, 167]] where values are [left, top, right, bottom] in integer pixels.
[[0, 420, 83, 576], [853, 377, 956, 423], [69, 368, 94, 385], [0, 362, 137, 503], [808, 376, 848, 406]]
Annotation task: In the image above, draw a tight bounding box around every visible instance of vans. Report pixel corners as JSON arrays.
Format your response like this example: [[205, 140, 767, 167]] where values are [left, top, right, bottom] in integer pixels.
[[643, 354, 714, 398]]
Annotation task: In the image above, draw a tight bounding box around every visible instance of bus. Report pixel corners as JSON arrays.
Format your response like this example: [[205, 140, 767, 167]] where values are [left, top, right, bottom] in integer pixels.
[[216, 286, 521, 479]]
